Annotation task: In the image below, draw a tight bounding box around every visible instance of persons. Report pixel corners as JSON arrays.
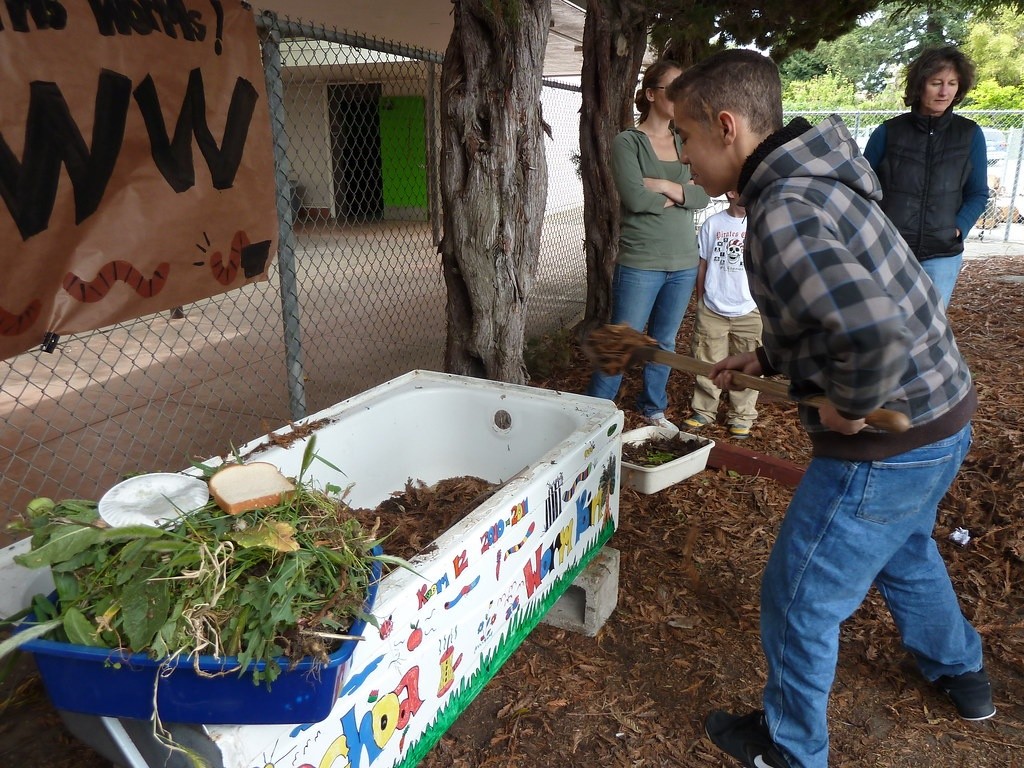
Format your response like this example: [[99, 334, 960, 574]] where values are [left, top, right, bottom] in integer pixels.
[[588, 57, 711, 432], [668, 49, 996, 768], [682, 191, 764, 441], [863, 47, 989, 316]]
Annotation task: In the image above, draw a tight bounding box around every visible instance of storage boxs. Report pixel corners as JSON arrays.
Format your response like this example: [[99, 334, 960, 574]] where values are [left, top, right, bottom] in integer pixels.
[[21, 542, 386, 725], [620, 426, 716, 495]]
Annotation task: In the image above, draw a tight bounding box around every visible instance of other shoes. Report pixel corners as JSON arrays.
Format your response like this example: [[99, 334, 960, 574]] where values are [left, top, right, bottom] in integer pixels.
[[728, 424, 749, 439], [642, 412, 680, 431], [681, 413, 708, 431]]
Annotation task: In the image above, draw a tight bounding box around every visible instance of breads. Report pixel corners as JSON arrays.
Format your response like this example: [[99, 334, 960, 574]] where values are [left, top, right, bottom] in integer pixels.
[[209, 462, 295, 516]]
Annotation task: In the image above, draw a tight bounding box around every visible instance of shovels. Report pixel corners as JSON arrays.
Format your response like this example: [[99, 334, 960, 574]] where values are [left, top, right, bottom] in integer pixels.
[[578, 320, 914, 436]]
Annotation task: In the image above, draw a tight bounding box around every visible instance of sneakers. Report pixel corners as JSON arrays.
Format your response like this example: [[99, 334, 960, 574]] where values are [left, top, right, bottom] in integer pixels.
[[939, 668, 997, 721], [705, 709, 790, 768]]
